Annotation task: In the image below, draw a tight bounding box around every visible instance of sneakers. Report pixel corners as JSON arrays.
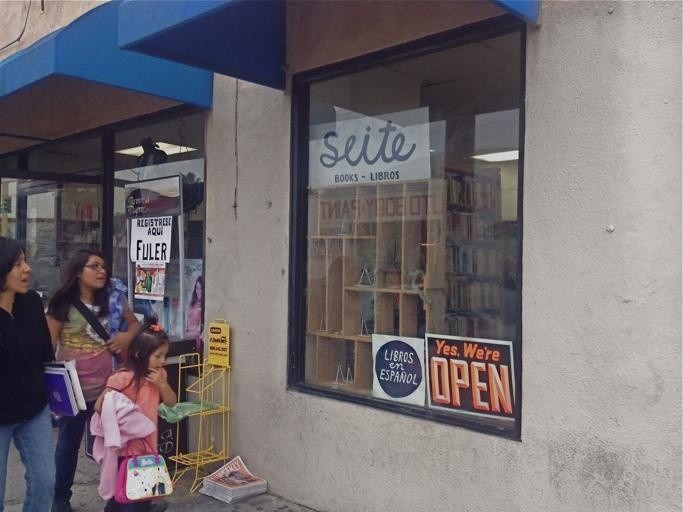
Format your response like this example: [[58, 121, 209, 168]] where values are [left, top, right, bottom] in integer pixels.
[[51, 496, 71, 512]]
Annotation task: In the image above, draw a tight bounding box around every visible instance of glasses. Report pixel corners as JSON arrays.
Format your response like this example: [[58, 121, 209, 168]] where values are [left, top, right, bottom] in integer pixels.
[[83, 264, 107, 270]]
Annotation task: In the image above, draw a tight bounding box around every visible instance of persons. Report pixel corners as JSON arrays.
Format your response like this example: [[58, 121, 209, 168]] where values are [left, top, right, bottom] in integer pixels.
[[45, 248, 140, 512], [0, 235, 59, 512], [187, 275, 203, 326], [92, 324, 178, 511]]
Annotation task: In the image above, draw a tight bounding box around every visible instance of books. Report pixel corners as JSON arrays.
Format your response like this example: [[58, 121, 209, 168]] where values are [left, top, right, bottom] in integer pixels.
[[42, 359, 87, 417], [198, 455, 267, 505], [447, 174, 502, 339]]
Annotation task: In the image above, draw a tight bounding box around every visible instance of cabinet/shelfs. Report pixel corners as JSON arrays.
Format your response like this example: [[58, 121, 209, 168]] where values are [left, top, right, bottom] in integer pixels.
[[431, 152, 504, 338], [500, 218, 521, 346], [167, 319, 231, 496], [305, 178, 447, 400]]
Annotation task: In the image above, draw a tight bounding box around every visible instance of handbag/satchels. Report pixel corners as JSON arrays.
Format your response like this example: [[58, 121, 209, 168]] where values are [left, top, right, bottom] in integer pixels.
[[114, 437, 173, 504]]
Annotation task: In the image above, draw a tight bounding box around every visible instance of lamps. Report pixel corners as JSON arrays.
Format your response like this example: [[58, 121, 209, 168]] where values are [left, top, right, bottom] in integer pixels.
[[137, 137, 168, 167]]
